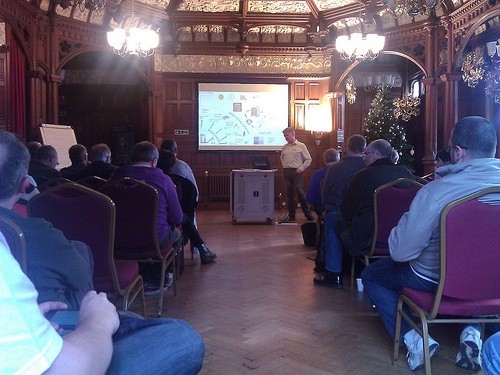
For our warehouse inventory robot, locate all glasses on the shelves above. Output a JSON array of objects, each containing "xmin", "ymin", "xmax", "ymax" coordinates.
[
  {"xmin": 362, "ymin": 152, "xmax": 379, "ymax": 156},
  {"xmin": 55, "ymin": 162, "xmax": 60, "ymax": 166}
]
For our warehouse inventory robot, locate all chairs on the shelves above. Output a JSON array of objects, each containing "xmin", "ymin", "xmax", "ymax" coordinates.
[
  {"xmin": 349, "ymin": 177, "xmax": 426, "ymax": 295},
  {"xmin": 24, "ymin": 181, "xmax": 146, "ymax": 322},
  {"xmin": 392, "ymin": 186, "xmax": 500, "ymax": 375},
  {"xmin": 167, "ymin": 173, "xmax": 200, "ymax": 279},
  {"xmin": 100, "ymin": 176, "xmax": 180, "ymax": 318}
]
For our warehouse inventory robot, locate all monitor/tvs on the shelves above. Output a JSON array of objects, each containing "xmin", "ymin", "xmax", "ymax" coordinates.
[{"xmin": 251, "ymin": 156, "xmax": 272, "ymax": 170}]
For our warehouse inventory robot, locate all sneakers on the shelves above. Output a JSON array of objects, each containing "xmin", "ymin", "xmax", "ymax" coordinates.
[
  {"xmin": 144, "ymin": 272, "xmax": 173, "ymax": 296},
  {"xmin": 403, "ymin": 333, "xmax": 440, "ymax": 370},
  {"xmin": 455, "ymin": 326, "xmax": 482, "ymax": 371}
]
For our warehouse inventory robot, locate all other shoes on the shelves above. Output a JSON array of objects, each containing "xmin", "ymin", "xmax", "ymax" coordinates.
[{"xmin": 307, "ymin": 250, "xmax": 316, "ymax": 260}]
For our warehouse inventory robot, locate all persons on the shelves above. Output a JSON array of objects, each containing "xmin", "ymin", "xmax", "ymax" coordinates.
[
  {"xmin": 25, "ymin": 146, "xmax": 63, "ymax": 192},
  {"xmin": 312, "ymin": 134, "xmax": 369, "ymax": 288},
  {"xmin": 57, "ymin": 144, "xmax": 92, "ymax": 185},
  {"xmin": 335, "ymin": 138, "xmax": 416, "ymax": 289},
  {"xmin": 434, "ymin": 146, "xmax": 453, "ymax": 172},
  {"xmin": 391, "ymin": 150, "xmax": 400, "ymax": 166},
  {"xmin": 75, "ymin": 144, "xmax": 121, "ymax": 185},
  {"xmin": 360, "ymin": 116, "xmax": 500, "ymax": 370},
  {"xmin": 277, "ymin": 126, "xmax": 315, "ymax": 223},
  {"xmin": 481, "ymin": 332, "xmax": 500, "ymax": 375},
  {"xmin": 306, "ymin": 147, "xmax": 341, "ymax": 253},
  {"xmin": 0, "ymin": 130, "xmax": 206, "ymax": 375},
  {"xmin": 25, "ymin": 141, "xmax": 42, "ymax": 157},
  {"xmin": 107, "ymin": 134, "xmax": 218, "ymax": 269}
]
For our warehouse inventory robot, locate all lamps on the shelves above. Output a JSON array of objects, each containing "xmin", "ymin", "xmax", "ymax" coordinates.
[
  {"xmin": 105, "ymin": 0, "xmax": 161, "ymax": 58},
  {"xmin": 334, "ymin": 0, "xmax": 386, "ymax": 62}
]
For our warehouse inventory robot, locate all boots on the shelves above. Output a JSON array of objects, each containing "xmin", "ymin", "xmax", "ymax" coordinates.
[{"xmin": 198, "ymin": 242, "xmax": 217, "ymax": 264}]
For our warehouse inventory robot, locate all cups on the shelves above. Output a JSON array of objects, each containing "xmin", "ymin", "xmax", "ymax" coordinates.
[{"xmin": 356, "ymin": 278, "xmax": 365, "ymax": 291}]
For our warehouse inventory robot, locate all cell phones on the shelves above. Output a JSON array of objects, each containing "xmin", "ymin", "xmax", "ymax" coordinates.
[{"xmin": 43, "ymin": 309, "xmax": 79, "ymax": 330}]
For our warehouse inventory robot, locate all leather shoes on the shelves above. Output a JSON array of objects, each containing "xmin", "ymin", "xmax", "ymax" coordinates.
[
  {"xmin": 314, "ymin": 275, "xmax": 344, "ymax": 289},
  {"xmin": 282, "ymin": 214, "xmax": 316, "ymax": 221}
]
